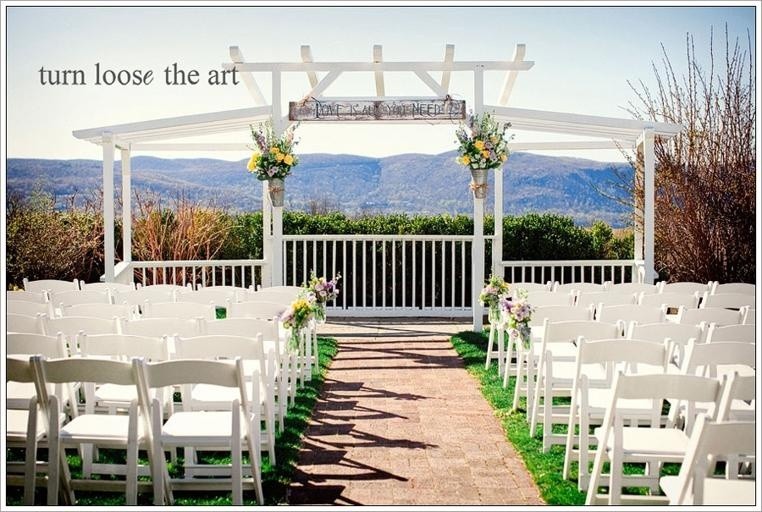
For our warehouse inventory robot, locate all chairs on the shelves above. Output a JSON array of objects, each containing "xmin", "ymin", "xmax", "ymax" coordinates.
[
  {"xmin": 6, "ymin": 355, "xmax": 77, "ymax": 505},
  {"xmin": 573, "ymin": 289, "xmax": 635, "ymax": 307},
  {"xmin": 23, "ymin": 277, "xmax": 79, "ymax": 291},
  {"xmin": 7, "ymin": 311, "xmax": 45, "ymax": 333},
  {"xmin": 115, "ymin": 290, "xmax": 172, "ymax": 306},
  {"xmin": 54, "ymin": 287, "xmax": 111, "ymax": 306},
  {"xmin": 638, "ymin": 291, "xmax": 700, "ymax": 309},
  {"xmin": 485, "ymin": 281, "xmax": 552, "ymax": 371},
  {"xmin": 584, "ymin": 372, "xmax": 723, "ymax": 506},
  {"xmin": 121, "ymin": 315, "xmax": 196, "ymax": 336},
  {"xmin": 228, "ymin": 298, "xmax": 297, "ymax": 408},
  {"xmin": 136, "ymin": 282, "xmax": 192, "ymax": 297},
  {"xmin": 513, "ymin": 304, "xmax": 595, "ymax": 421},
  {"xmin": 711, "ymin": 280, "xmax": 754, "ymax": 293},
  {"xmin": 37, "ymin": 353, "xmax": 174, "ymax": 504},
  {"xmin": 742, "ymin": 305, "xmax": 755, "ymax": 324},
  {"xmin": 239, "ymin": 292, "xmax": 307, "ymax": 390},
  {"xmin": 79, "ymin": 280, "xmax": 132, "ymax": 295},
  {"xmin": 145, "ymin": 299, "xmax": 217, "ymax": 318},
  {"xmin": 44, "ymin": 314, "xmax": 118, "ymax": 334},
  {"xmin": 6, "ymin": 332, "xmax": 67, "ymax": 361},
  {"xmin": 659, "ymin": 412, "xmax": 757, "ymax": 505},
  {"xmin": 529, "ymin": 319, "xmax": 623, "ymax": 454},
  {"xmin": 706, "ymin": 322, "xmax": 756, "ymax": 344},
  {"xmin": 61, "ymin": 301, "xmax": 136, "ymax": 317},
  {"xmin": 555, "ymin": 281, "xmax": 604, "ymax": 293},
  {"xmin": 719, "ymin": 370, "xmax": 757, "ymax": 478},
  {"xmin": 196, "ymin": 283, "xmax": 254, "ymax": 300},
  {"xmin": 630, "ymin": 319, "xmax": 701, "ymax": 361},
  {"xmin": 176, "ymin": 292, "xmax": 236, "ymax": 303},
  {"xmin": 701, "ymin": 291, "xmax": 755, "ymax": 309},
  {"xmin": 563, "ymin": 336, "xmax": 667, "ymax": 481},
  {"xmin": 598, "ymin": 302, "xmax": 668, "ymax": 320},
  {"xmin": 198, "ymin": 316, "xmax": 285, "ymax": 434},
  {"xmin": 79, "ymin": 331, "xmax": 177, "ymax": 477},
  {"xmin": 678, "ymin": 339, "xmax": 756, "ymax": 435},
  {"xmin": 132, "ymin": 357, "xmax": 264, "ymax": 505},
  {"xmin": 679, "ymin": 305, "xmax": 744, "ymax": 329},
  {"xmin": 498, "ymin": 291, "xmax": 579, "ymax": 389},
  {"xmin": 8, "ymin": 291, "xmax": 51, "ymax": 316},
  {"xmin": 258, "ymin": 284, "xmax": 320, "ymax": 382},
  {"xmin": 660, "ymin": 280, "xmax": 713, "ymax": 295},
  {"xmin": 174, "ymin": 334, "xmax": 276, "ymax": 467},
  {"xmin": 607, "ymin": 280, "xmax": 662, "ymax": 293}
]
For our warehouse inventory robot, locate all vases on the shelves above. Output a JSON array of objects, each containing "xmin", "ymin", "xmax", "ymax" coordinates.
[
  {"xmin": 270, "ymin": 177, "xmax": 286, "ymax": 208},
  {"xmin": 472, "ymin": 169, "xmax": 488, "ymax": 198}
]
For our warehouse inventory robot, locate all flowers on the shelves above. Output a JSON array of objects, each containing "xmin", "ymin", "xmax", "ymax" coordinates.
[
  {"xmin": 245, "ymin": 115, "xmax": 301, "ymax": 181},
  {"xmin": 455, "ymin": 108, "xmax": 515, "ymax": 169},
  {"xmin": 479, "ymin": 273, "xmax": 507, "ymax": 316},
  {"xmin": 282, "ymin": 298, "xmax": 324, "ymax": 350},
  {"xmin": 506, "ymin": 289, "xmax": 534, "ymax": 347},
  {"xmin": 305, "ymin": 272, "xmax": 341, "ymax": 319}
]
